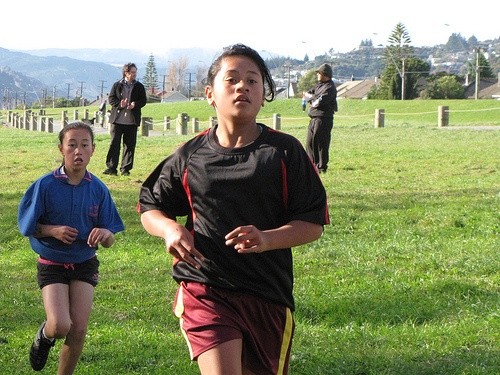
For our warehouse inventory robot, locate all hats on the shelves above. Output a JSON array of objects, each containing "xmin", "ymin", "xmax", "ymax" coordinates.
[{"xmin": 315, "ymin": 64, "xmax": 332, "ymax": 77}]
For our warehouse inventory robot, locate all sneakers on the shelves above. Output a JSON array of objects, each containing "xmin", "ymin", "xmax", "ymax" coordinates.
[{"xmin": 30, "ymin": 322, "xmax": 56, "ymax": 371}]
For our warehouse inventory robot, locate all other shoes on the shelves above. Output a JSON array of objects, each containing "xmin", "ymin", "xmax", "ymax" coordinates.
[
  {"xmin": 102, "ymin": 169, "xmax": 118, "ymax": 174},
  {"xmin": 121, "ymin": 171, "xmax": 130, "ymax": 176}
]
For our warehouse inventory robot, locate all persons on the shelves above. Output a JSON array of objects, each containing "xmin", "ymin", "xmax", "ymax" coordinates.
[
  {"xmin": 17, "ymin": 120, "xmax": 125, "ymax": 375},
  {"xmin": 303, "ymin": 64, "xmax": 338, "ymax": 172},
  {"xmin": 102, "ymin": 62, "xmax": 147, "ymax": 176},
  {"xmin": 138, "ymin": 44, "xmax": 331, "ymax": 375}
]
